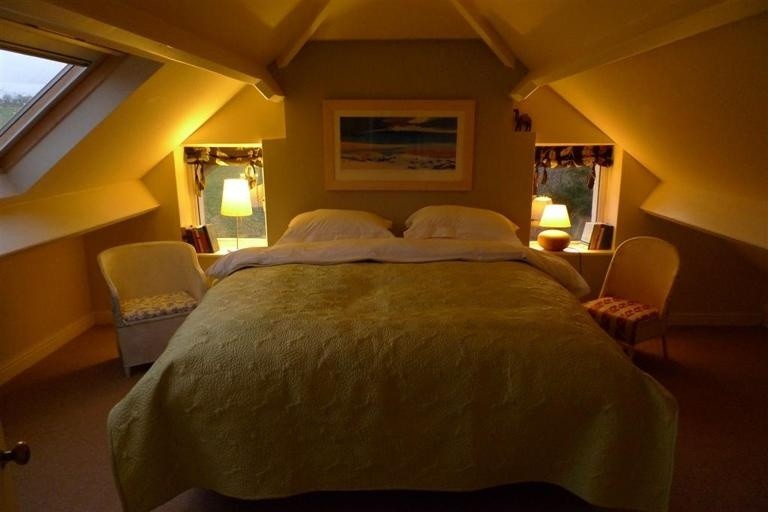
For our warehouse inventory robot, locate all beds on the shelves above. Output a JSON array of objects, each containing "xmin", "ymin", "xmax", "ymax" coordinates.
[{"xmin": 107, "ymin": 205, "xmax": 680, "ymax": 512}]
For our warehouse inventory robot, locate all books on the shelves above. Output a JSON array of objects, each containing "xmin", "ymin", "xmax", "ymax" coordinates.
[
  {"xmin": 578, "ymin": 221, "xmax": 614, "ymax": 250},
  {"xmin": 180, "ymin": 224, "xmax": 220, "ymax": 253}
]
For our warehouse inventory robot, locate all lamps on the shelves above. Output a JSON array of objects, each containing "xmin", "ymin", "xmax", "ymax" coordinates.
[
  {"xmin": 531, "ymin": 197, "xmax": 553, "ymax": 239},
  {"xmin": 221, "ymin": 178, "xmax": 253, "ymax": 252},
  {"xmin": 537, "ymin": 205, "xmax": 572, "ymax": 251}
]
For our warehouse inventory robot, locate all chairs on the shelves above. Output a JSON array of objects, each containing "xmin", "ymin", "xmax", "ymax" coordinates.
[
  {"xmin": 582, "ymin": 236, "xmax": 680, "ymax": 362},
  {"xmin": 96, "ymin": 241, "xmax": 209, "ymax": 378}
]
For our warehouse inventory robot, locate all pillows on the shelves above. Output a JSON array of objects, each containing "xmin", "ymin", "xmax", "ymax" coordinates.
[
  {"xmin": 403, "ymin": 205, "xmax": 523, "ymax": 241},
  {"xmin": 274, "ymin": 208, "xmax": 396, "ymax": 245}
]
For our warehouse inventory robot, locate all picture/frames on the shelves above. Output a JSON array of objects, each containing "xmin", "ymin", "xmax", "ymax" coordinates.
[{"xmin": 322, "ymin": 99, "xmax": 476, "ymax": 192}]
